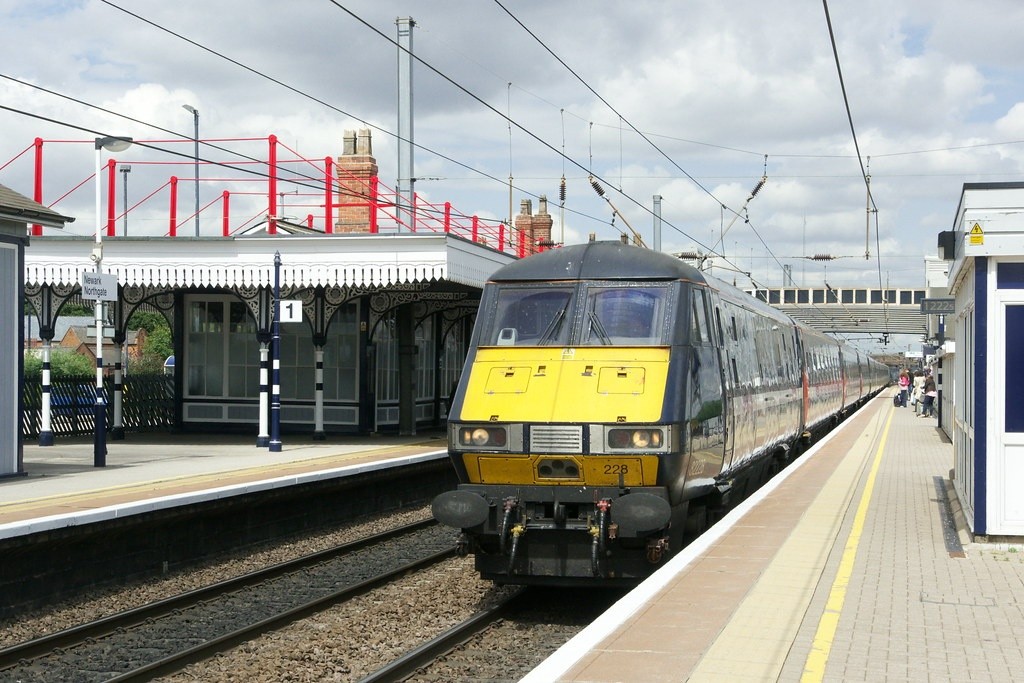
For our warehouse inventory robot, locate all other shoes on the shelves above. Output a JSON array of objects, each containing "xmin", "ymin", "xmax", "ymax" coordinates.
[{"xmin": 918, "ymin": 414, "xmax": 926, "ymax": 417}]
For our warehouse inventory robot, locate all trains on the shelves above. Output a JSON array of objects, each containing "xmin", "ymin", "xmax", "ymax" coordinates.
[{"xmin": 430, "ymin": 232, "xmax": 895, "ymax": 588}]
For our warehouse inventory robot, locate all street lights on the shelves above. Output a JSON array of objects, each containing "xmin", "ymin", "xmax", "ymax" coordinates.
[
  {"xmin": 91, "ymin": 136, "xmax": 133, "ymax": 468},
  {"xmin": 182, "ymin": 102, "xmax": 200, "ymax": 236}
]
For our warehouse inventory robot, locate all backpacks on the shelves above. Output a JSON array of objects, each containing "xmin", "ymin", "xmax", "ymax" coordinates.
[{"xmin": 894, "ymin": 396, "xmax": 900, "ymax": 407}]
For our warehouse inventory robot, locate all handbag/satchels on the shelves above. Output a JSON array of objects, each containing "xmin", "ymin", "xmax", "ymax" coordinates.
[
  {"xmin": 926, "ymin": 391, "xmax": 937, "ymax": 397},
  {"xmin": 899, "ymin": 383, "xmax": 908, "ymax": 390}
]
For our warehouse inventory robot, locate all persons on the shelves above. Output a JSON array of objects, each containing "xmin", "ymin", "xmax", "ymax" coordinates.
[
  {"xmin": 919, "ymin": 375, "xmax": 937, "ymax": 419},
  {"xmin": 898, "ymin": 368, "xmax": 914, "ymax": 407},
  {"xmin": 910, "ymin": 367, "xmax": 932, "ymax": 411}
]
